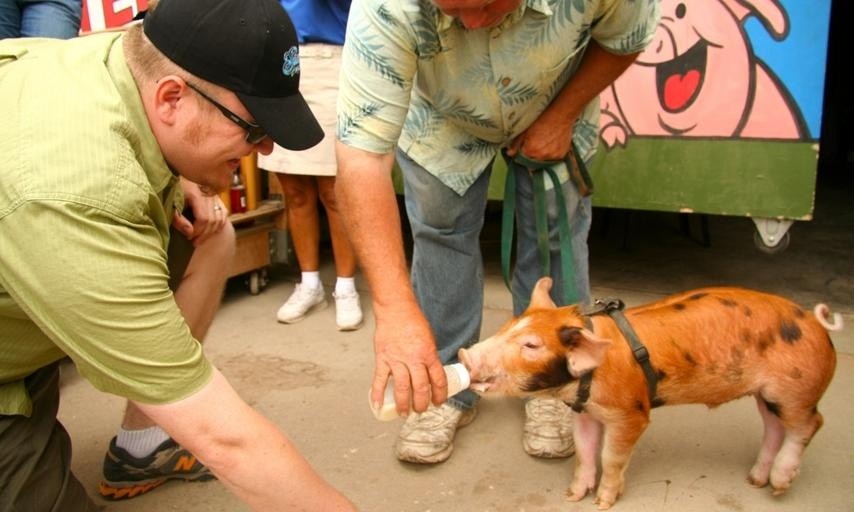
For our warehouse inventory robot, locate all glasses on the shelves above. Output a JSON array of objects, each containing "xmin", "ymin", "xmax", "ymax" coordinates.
[{"xmin": 185, "ymin": 85, "xmax": 268, "ymax": 145}]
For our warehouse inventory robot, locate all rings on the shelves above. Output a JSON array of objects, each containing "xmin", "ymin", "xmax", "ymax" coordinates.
[{"xmin": 213, "ymin": 206, "xmax": 221, "ymax": 210}]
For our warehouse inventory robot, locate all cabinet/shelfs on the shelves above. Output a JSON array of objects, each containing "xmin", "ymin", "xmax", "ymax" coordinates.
[{"xmin": 167, "ymin": 143, "xmax": 286, "ymax": 293}]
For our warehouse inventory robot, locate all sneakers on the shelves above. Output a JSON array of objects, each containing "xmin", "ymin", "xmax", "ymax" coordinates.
[
  {"xmin": 333, "ymin": 282, "xmax": 365, "ymax": 331},
  {"xmin": 99, "ymin": 434, "xmax": 221, "ymax": 500},
  {"xmin": 524, "ymin": 399, "xmax": 576, "ymax": 459},
  {"xmin": 277, "ymin": 280, "xmax": 329, "ymax": 324},
  {"xmin": 395, "ymin": 402, "xmax": 478, "ymax": 464}
]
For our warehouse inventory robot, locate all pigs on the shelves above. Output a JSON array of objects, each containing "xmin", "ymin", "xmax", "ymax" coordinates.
[{"xmin": 457, "ymin": 276, "xmax": 845, "ymax": 510}]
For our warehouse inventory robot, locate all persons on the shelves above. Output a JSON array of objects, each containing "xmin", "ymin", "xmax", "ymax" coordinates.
[
  {"xmin": 0, "ymin": 1, "xmax": 82, "ymax": 40},
  {"xmin": 0, "ymin": 1, "xmax": 363, "ymax": 511},
  {"xmin": 331, "ymin": 0, "xmax": 660, "ymax": 466},
  {"xmin": 257, "ymin": 1, "xmax": 364, "ymax": 331}
]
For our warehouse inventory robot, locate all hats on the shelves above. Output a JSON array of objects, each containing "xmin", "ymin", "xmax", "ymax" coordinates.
[{"xmin": 142, "ymin": 1, "xmax": 324, "ymax": 150}]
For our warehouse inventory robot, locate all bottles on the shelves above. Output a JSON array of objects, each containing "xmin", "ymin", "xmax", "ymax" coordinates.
[
  {"xmin": 230, "ymin": 185, "xmax": 249, "ymax": 216},
  {"xmin": 365, "ymin": 360, "xmax": 473, "ymax": 422}
]
